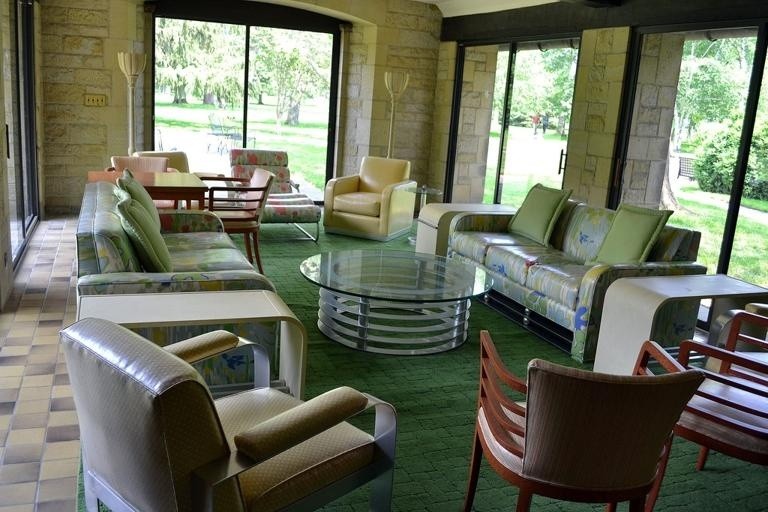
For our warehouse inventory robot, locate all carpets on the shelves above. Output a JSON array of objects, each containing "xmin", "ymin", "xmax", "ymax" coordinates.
[{"xmin": 76, "ymin": 211, "xmax": 768, "ymax": 512}]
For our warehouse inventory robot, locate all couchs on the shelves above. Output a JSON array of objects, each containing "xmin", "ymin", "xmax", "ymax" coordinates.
[
  {"xmin": 322, "ymin": 156, "xmax": 418, "ymax": 242},
  {"xmin": 133, "ymin": 151, "xmax": 224, "ymax": 209},
  {"xmin": 75, "ymin": 181, "xmax": 279, "ymax": 386},
  {"xmin": 417, "ymin": 197, "xmax": 708, "ymax": 365}
]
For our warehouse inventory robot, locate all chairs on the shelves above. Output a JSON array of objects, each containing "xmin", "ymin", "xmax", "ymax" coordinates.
[
  {"xmin": 194, "ymin": 167, "xmax": 276, "ymax": 274},
  {"xmin": 704, "ymin": 303, "xmax": 768, "ymax": 373},
  {"xmin": 228, "ymin": 148, "xmax": 321, "ymax": 242},
  {"xmin": 645, "ymin": 312, "xmax": 768, "ymax": 511},
  {"xmin": 57, "ymin": 316, "xmax": 398, "ymax": 512},
  {"xmin": 106, "ymin": 156, "xmax": 181, "ymax": 209},
  {"xmin": 462, "ymin": 329, "xmax": 705, "ymax": 512}
]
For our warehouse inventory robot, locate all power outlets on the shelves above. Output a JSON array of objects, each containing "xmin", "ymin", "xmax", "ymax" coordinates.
[{"xmin": 85, "ymin": 94, "xmax": 106, "ymax": 107}]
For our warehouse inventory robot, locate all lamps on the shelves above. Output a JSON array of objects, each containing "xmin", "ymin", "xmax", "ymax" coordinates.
[
  {"xmin": 384, "ymin": 71, "xmax": 410, "ymax": 159},
  {"xmin": 118, "ymin": 52, "xmax": 148, "ymax": 157}
]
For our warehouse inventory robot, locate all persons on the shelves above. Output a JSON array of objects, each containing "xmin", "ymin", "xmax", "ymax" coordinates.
[
  {"xmin": 540, "ymin": 113, "xmax": 549, "ymax": 134},
  {"xmin": 531, "ymin": 112, "xmax": 540, "ymax": 135}
]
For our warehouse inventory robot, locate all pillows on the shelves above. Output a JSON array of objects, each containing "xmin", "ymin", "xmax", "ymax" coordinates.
[
  {"xmin": 507, "ymin": 183, "xmax": 574, "ymax": 247},
  {"xmin": 584, "ymin": 202, "xmax": 674, "ymax": 267},
  {"xmin": 116, "ymin": 168, "xmax": 162, "ymax": 232},
  {"xmin": 113, "ymin": 184, "xmax": 173, "ymax": 273}
]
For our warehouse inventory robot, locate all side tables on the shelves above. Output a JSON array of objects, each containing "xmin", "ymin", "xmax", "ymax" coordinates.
[
  {"xmin": 404, "ymin": 184, "xmax": 442, "ymax": 245},
  {"xmin": 75, "ymin": 289, "xmax": 308, "ymax": 400},
  {"xmin": 593, "ymin": 273, "xmax": 768, "ymax": 376}
]
